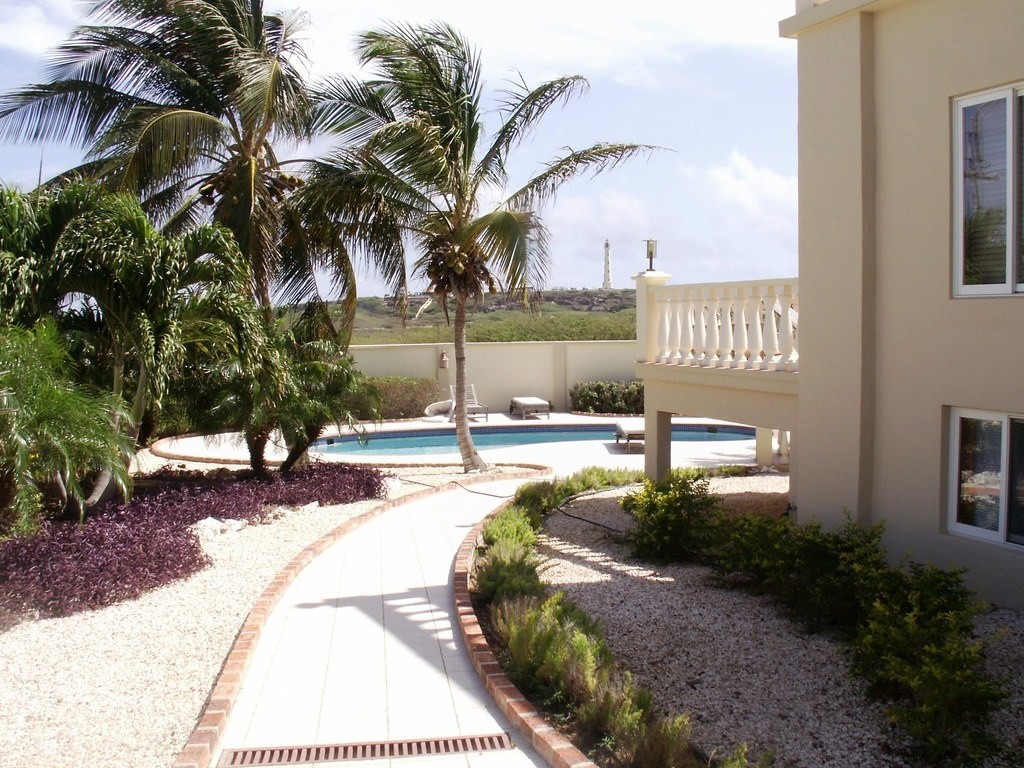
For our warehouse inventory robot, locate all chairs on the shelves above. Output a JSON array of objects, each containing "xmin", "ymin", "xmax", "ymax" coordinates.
[{"xmin": 447, "ymin": 382, "xmax": 489, "ymax": 422}]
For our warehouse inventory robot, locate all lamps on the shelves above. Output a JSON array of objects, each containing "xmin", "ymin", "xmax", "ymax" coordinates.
[{"xmin": 644, "ymin": 237, "xmax": 659, "ymax": 272}]
